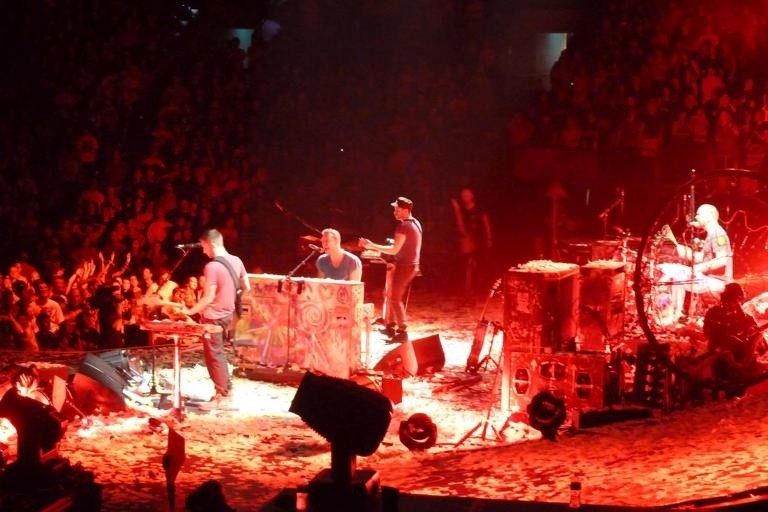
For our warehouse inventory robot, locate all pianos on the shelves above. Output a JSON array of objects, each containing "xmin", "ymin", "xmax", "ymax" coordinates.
[
  {"xmin": 139, "ymin": 321, "xmax": 223, "ymax": 334},
  {"xmin": 232, "ymin": 274, "xmax": 375, "ymax": 380}
]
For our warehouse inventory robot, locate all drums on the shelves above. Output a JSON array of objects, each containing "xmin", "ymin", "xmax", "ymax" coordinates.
[
  {"xmin": 594, "ymin": 240, "xmax": 620, "ymax": 263},
  {"xmin": 566, "ymin": 242, "xmax": 591, "ymax": 276}
]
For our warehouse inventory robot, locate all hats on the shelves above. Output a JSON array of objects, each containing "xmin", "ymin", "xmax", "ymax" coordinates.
[{"xmin": 390, "ymin": 197, "xmax": 412, "ymax": 210}]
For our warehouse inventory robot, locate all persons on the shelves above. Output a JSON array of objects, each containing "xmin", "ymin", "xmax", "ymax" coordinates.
[
  {"xmin": 702, "ymin": 281, "xmax": 768, "ymax": 392},
  {"xmin": 179, "ymin": 227, "xmax": 251, "ymax": 412},
  {"xmin": 0, "ymin": 0, "xmax": 768, "ymax": 354}
]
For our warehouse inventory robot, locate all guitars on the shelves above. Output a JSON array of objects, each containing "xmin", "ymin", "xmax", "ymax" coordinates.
[
  {"xmin": 450, "ymin": 197, "xmax": 485, "ymax": 263},
  {"xmin": 467, "ymin": 278, "xmax": 502, "ymax": 375}
]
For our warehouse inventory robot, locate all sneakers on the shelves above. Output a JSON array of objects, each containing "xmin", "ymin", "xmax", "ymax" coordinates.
[
  {"xmin": 198, "ymin": 393, "xmax": 231, "ymax": 411},
  {"xmin": 378, "ymin": 328, "xmax": 394, "ymax": 337},
  {"xmin": 386, "ymin": 332, "xmax": 408, "ymax": 343}
]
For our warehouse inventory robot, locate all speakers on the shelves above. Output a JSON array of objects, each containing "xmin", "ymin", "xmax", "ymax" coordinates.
[
  {"xmin": 98, "ymin": 349, "xmax": 129, "ymax": 369},
  {"xmin": 372, "ymin": 332, "xmax": 445, "ymax": 376},
  {"xmin": 73, "ymin": 353, "xmax": 124, "ymax": 396}
]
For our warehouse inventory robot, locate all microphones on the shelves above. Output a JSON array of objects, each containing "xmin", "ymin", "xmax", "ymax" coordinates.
[
  {"xmin": 175, "ymin": 241, "xmax": 202, "ymax": 251},
  {"xmin": 309, "ymin": 241, "xmax": 327, "ymax": 258}
]
